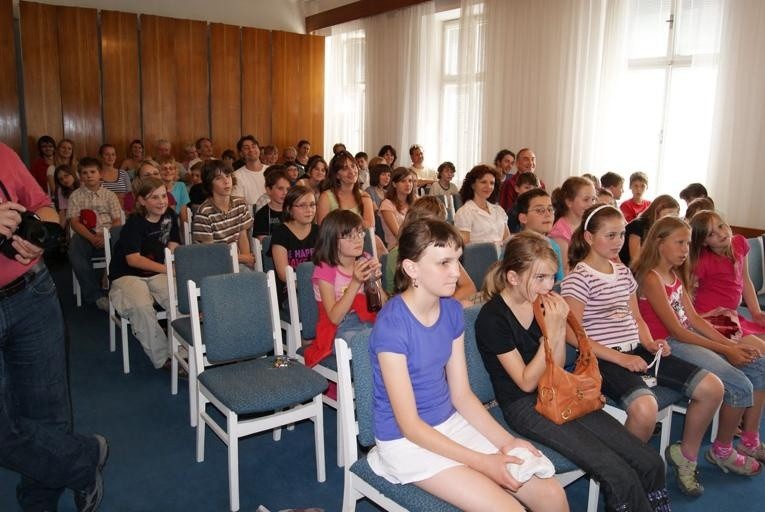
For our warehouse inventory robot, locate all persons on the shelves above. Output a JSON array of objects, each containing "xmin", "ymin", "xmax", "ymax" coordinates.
[
  {"xmin": 473, "ymin": 229, "xmax": 670, "ymax": 512},
  {"xmin": 686, "ymin": 209, "xmax": 765, "ymax": 358},
  {"xmin": 1, "ymin": 143, "xmax": 109, "ymax": 512},
  {"xmin": 303, "ymin": 162, "xmax": 712, "ymax": 411},
  {"xmin": 562, "ymin": 203, "xmax": 724, "ymax": 496},
  {"xmin": 32, "ymin": 135, "xmax": 546, "ymax": 373},
  {"xmin": 632, "ymin": 217, "xmax": 765, "ymax": 479},
  {"xmin": 364, "ymin": 217, "xmax": 571, "ymax": 512}
]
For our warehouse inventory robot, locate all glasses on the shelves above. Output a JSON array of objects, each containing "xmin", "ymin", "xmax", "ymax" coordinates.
[
  {"xmin": 338, "ymin": 232, "xmax": 366, "ymax": 241},
  {"xmin": 525, "ymin": 208, "xmax": 559, "ymax": 216},
  {"xmin": 290, "ymin": 202, "xmax": 318, "ymax": 210}
]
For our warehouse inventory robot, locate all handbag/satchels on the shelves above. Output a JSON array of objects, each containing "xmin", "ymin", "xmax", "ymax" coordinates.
[{"xmin": 534, "ymin": 352, "xmax": 606, "ymax": 425}]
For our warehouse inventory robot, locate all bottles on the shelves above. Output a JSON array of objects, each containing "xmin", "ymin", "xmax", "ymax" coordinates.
[{"xmin": 364, "ymin": 266, "xmax": 382, "ymax": 312}]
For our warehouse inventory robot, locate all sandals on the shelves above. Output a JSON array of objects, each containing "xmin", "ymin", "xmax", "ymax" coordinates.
[
  {"xmin": 706, "ymin": 444, "xmax": 760, "ymax": 477},
  {"xmin": 737, "ymin": 438, "xmax": 765, "ymax": 459}
]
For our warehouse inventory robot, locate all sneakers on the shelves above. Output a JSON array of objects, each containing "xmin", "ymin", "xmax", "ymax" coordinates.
[
  {"xmin": 164, "ymin": 357, "xmax": 187, "ymax": 377},
  {"xmin": 663, "ymin": 440, "xmax": 706, "ymax": 498},
  {"xmin": 74, "ymin": 433, "xmax": 107, "ymax": 512},
  {"xmin": 95, "ymin": 296, "xmax": 110, "ymax": 313}
]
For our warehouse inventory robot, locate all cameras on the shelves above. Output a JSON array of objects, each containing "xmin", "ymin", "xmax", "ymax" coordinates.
[{"xmin": 0, "ymin": 210, "xmax": 64, "ymax": 261}]
